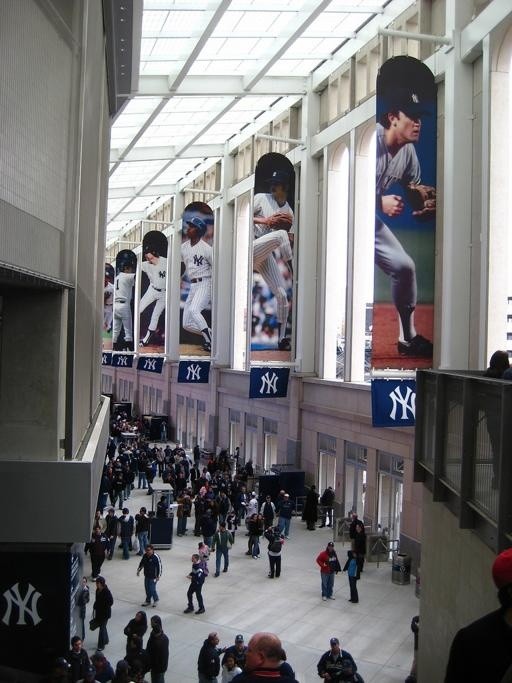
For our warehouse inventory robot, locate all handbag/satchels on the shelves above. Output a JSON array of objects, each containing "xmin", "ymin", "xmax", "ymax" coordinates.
[
  {"xmin": 133, "ymin": 538, "xmax": 140, "ymax": 552},
  {"xmin": 90, "ymin": 617, "xmax": 100, "ymax": 630}
]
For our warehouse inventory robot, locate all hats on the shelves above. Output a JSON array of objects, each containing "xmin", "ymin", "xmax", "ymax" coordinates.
[
  {"xmin": 328, "ymin": 541, "xmax": 334, "ymax": 546},
  {"xmin": 236, "ymin": 634, "xmax": 244, "ymax": 642},
  {"xmin": 265, "ymin": 168, "xmax": 290, "ymax": 185},
  {"xmin": 491, "ymin": 549, "xmax": 512, "ymax": 587},
  {"xmin": 390, "ymin": 84, "xmax": 433, "ymax": 116},
  {"xmin": 330, "ymin": 637, "xmax": 339, "ymax": 644},
  {"xmin": 55, "ymin": 657, "xmax": 72, "ymax": 669}
]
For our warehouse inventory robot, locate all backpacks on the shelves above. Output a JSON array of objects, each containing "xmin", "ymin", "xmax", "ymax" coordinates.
[{"xmin": 269, "ymin": 533, "xmax": 281, "ymax": 552}]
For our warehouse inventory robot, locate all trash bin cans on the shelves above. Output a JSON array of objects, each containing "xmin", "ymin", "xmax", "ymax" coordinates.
[
  {"xmin": 391, "ymin": 553, "xmax": 411, "ymax": 584},
  {"xmin": 415, "ymin": 567, "xmax": 422, "ymax": 598},
  {"xmin": 411, "ymin": 616, "xmax": 419, "ymax": 658}
]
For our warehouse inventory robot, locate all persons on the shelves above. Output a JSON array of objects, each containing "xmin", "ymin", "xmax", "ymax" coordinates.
[
  {"xmin": 220, "ymin": 632, "xmax": 250, "ymax": 672},
  {"xmin": 90, "ymin": 576, "xmax": 114, "ymax": 650},
  {"xmin": 80, "ymin": 576, "xmax": 89, "ymax": 642},
  {"xmin": 134, "ymin": 544, "xmax": 163, "ymax": 607},
  {"xmin": 113, "ymin": 260, "xmax": 135, "ymax": 351},
  {"xmin": 302, "ymin": 484, "xmax": 319, "ymax": 530},
  {"xmin": 141, "ymin": 246, "xmax": 166, "ymax": 346},
  {"xmin": 251, "ymin": 171, "xmax": 296, "ymax": 354},
  {"xmin": 182, "ymin": 216, "xmax": 212, "ymax": 353},
  {"xmin": 316, "ymin": 638, "xmax": 364, "ymax": 682},
  {"xmin": 218, "ymin": 652, "xmax": 243, "ymax": 682},
  {"xmin": 50, "ymin": 612, "xmax": 169, "ymax": 682},
  {"xmin": 182, "ymin": 554, "xmax": 208, "ymax": 614},
  {"xmin": 104, "ymin": 270, "xmax": 113, "ymax": 330},
  {"xmin": 444, "ymin": 547, "xmax": 512, "ymax": 682},
  {"xmin": 197, "ymin": 631, "xmax": 228, "ymax": 682},
  {"xmin": 372, "ymin": 90, "xmax": 436, "ymax": 361},
  {"xmin": 229, "ymin": 631, "xmax": 298, "ymax": 682},
  {"xmin": 355, "ymin": 523, "xmax": 365, "ymax": 572},
  {"xmin": 315, "ymin": 542, "xmax": 341, "ymax": 600},
  {"xmin": 319, "ymin": 486, "xmax": 334, "ymax": 528},
  {"xmin": 349, "ymin": 512, "xmax": 365, "ymax": 549},
  {"xmin": 343, "ymin": 549, "xmax": 358, "ymax": 603},
  {"xmin": 481, "ymin": 350, "xmax": 512, "ymax": 491},
  {"xmin": 87, "ymin": 413, "xmax": 294, "ymax": 582}
]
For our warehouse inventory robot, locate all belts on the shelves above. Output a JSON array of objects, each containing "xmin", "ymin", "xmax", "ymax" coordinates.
[
  {"xmin": 116, "ymin": 300, "xmax": 126, "ymax": 303},
  {"xmin": 106, "ymin": 304, "xmax": 113, "ymax": 305},
  {"xmin": 152, "ymin": 285, "xmax": 166, "ymax": 291},
  {"xmin": 191, "ymin": 277, "xmax": 203, "ymax": 282}
]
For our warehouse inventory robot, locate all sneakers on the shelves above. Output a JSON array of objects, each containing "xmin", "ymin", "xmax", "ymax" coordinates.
[
  {"xmin": 277, "ymin": 338, "xmax": 291, "ymax": 351},
  {"xmin": 97, "ymin": 646, "xmax": 105, "ymax": 650},
  {"xmin": 141, "ymin": 551, "xmax": 359, "ymax": 614},
  {"xmin": 113, "ymin": 340, "xmax": 211, "ymax": 351},
  {"xmin": 397, "ymin": 333, "xmax": 433, "ymax": 359}
]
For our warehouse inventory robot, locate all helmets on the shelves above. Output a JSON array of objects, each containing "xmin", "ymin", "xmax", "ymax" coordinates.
[
  {"xmin": 186, "ymin": 216, "xmax": 207, "ymax": 235},
  {"xmin": 143, "ymin": 245, "xmax": 154, "ymax": 254},
  {"xmin": 119, "ymin": 259, "xmax": 133, "ymax": 267},
  {"xmin": 105, "ymin": 262, "xmax": 115, "ymax": 279}
]
